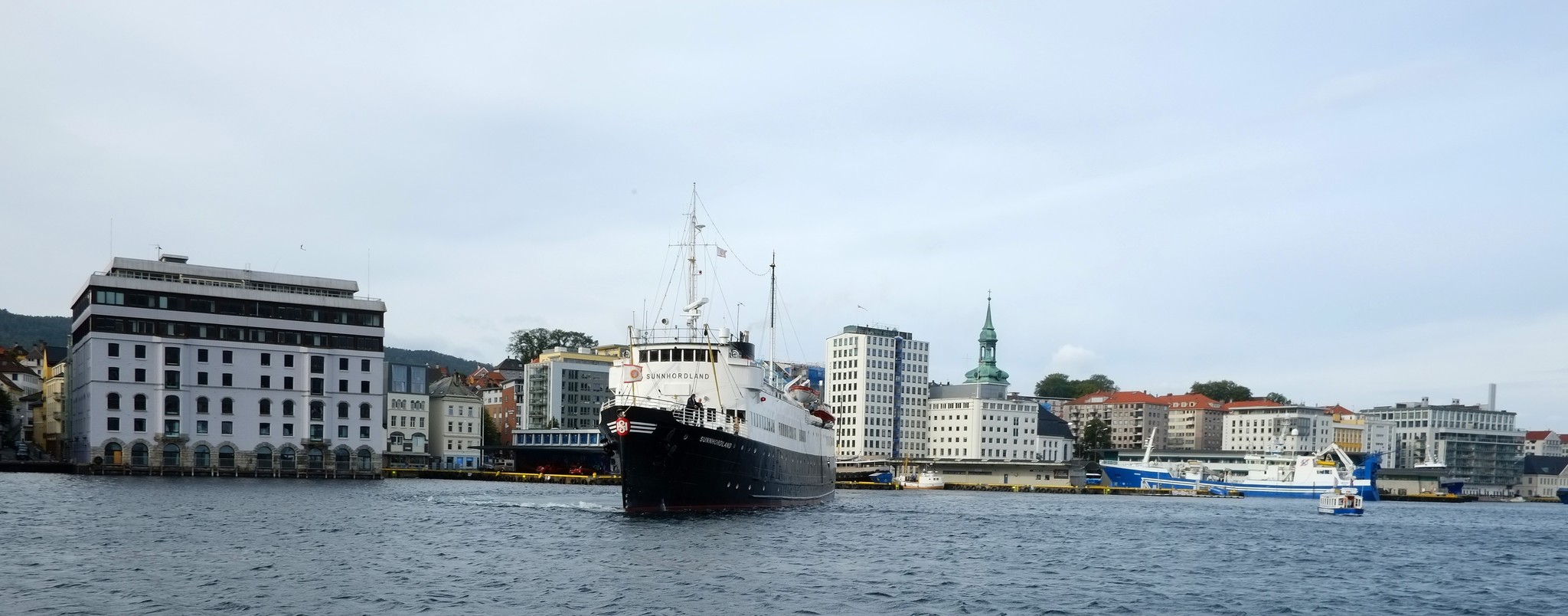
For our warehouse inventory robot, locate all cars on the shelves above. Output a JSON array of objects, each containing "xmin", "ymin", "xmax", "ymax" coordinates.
[
  {"xmin": 13, "ymin": 441, "xmax": 23, "ymax": 449},
  {"xmin": 478, "ymin": 464, "xmax": 494, "ymax": 471},
  {"xmin": 535, "ymin": 462, "xmax": 563, "ymax": 474},
  {"xmin": 16, "ymin": 444, "xmax": 28, "ymax": 451},
  {"xmin": 569, "ymin": 465, "xmax": 596, "ymax": 475},
  {"xmin": 16, "ymin": 449, "xmax": 29, "ymax": 460}
]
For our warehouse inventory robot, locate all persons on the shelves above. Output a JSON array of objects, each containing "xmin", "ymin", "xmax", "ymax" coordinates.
[
  {"xmin": 684, "ymin": 394, "xmax": 700, "ymax": 425},
  {"xmin": 694, "ymin": 398, "xmax": 704, "ymax": 428}
]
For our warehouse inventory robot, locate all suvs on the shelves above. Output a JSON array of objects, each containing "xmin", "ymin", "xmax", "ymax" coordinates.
[{"xmin": 494, "ymin": 462, "xmax": 513, "ymax": 472}]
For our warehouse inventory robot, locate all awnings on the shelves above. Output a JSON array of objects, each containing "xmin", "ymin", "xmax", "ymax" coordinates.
[{"xmin": 382, "ymin": 451, "xmax": 431, "ymax": 456}]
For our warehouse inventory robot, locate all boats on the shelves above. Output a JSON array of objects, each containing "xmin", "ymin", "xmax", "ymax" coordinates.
[
  {"xmin": 1414, "ymin": 444, "xmax": 1448, "ymax": 469},
  {"xmin": 788, "ymin": 385, "xmax": 819, "ymax": 405},
  {"xmin": 1317, "ymin": 476, "xmax": 1364, "ymax": 517},
  {"xmin": 1098, "ymin": 424, "xmax": 1383, "ymax": 501},
  {"xmin": 893, "ymin": 453, "xmax": 945, "ymax": 490},
  {"xmin": 811, "ymin": 409, "xmax": 836, "ymax": 425},
  {"xmin": 1510, "ymin": 496, "xmax": 1528, "ymax": 502},
  {"xmin": 1556, "ymin": 488, "xmax": 1568, "ymax": 504},
  {"xmin": 597, "ymin": 179, "xmax": 836, "ymax": 518},
  {"xmin": 1500, "ymin": 499, "xmax": 1511, "ymax": 502}
]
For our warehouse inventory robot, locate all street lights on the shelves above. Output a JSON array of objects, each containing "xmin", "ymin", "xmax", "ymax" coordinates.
[
  {"xmin": 1094, "ymin": 428, "xmax": 1108, "ymax": 462},
  {"xmin": 1079, "ymin": 425, "xmax": 1092, "ymax": 466}
]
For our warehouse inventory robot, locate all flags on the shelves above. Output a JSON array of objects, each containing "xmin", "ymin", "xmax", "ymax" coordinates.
[
  {"xmin": 717, "ymin": 247, "xmax": 727, "ymax": 258},
  {"xmin": 623, "ymin": 364, "xmax": 643, "ymax": 383}
]
